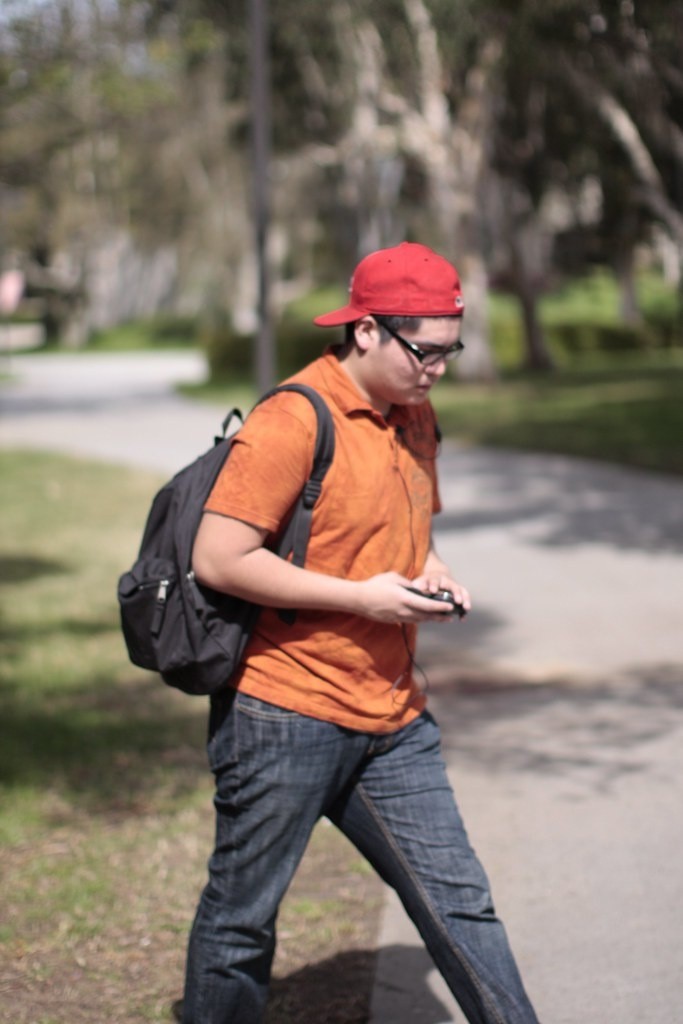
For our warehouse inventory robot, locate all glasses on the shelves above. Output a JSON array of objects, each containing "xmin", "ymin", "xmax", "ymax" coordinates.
[{"xmin": 375, "ymin": 319, "xmax": 464, "ymax": 366}]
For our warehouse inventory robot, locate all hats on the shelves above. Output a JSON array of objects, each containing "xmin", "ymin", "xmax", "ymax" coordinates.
[{"xmin": 314, "ymin": 242, "xmax": 464, "ymax": 326}]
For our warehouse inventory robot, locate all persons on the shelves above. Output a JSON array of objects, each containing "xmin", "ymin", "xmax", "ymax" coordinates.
[
  {"xmin": 173, "ymin": 244, "xmax": 539, "ymax": 1024},
  {"xmin": 24, "ymin": 248, "xmax": 67, "ymax": 337}
]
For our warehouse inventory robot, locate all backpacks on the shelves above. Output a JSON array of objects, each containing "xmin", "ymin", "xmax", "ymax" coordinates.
[{"xmin": 117, "ymin": 383, "xmax": 334, "ymax": 697}]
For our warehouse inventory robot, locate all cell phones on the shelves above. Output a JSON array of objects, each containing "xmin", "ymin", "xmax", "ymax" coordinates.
[{"xmin": 409, "ymin": 587, "xmax": 462, "ymax": 609}]
